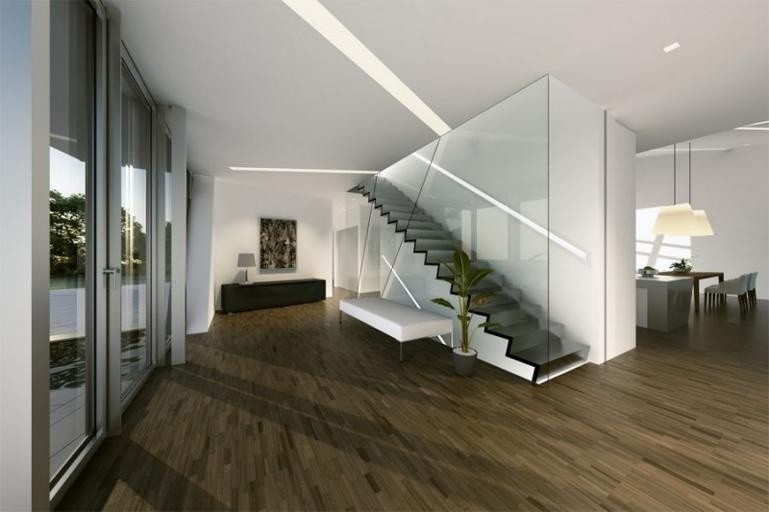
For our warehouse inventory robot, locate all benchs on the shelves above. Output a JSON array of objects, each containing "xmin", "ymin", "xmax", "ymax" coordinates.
[{"xmin": 337, "ymin": 295, "xmax": 455, "ymax": 361}]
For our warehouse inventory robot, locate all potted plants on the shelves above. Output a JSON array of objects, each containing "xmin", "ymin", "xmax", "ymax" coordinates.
[
  {"xmin": 668, "ymin": 257, "xmax": 692, "ymax": 274},
  {"xmin": 431, "ymin": 246, "xmax": 501, "ymax": 375}
]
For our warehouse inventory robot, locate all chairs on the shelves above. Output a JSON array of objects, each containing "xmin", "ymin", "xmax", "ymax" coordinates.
[{"xmin": 702, "ymin": 271, "xmax": 760, "ymax": 317}]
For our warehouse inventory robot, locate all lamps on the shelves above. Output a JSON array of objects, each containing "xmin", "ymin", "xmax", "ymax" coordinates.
[
  {"xmin": 236, "ymin": 253, "xmax": 258, "ymax": 286},
  {"xmin": 653, "ymin": 141, "xmax": 713, "ymax": 240}
]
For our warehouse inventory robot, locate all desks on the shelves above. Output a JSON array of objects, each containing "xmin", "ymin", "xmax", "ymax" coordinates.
[
  {"xmin": 658, "ymin": 271, "xmax": 726, "ymax": 313},
  {"xmin": 220, "ymin": 277, "xmax": 329, "ymax": 315}
]
